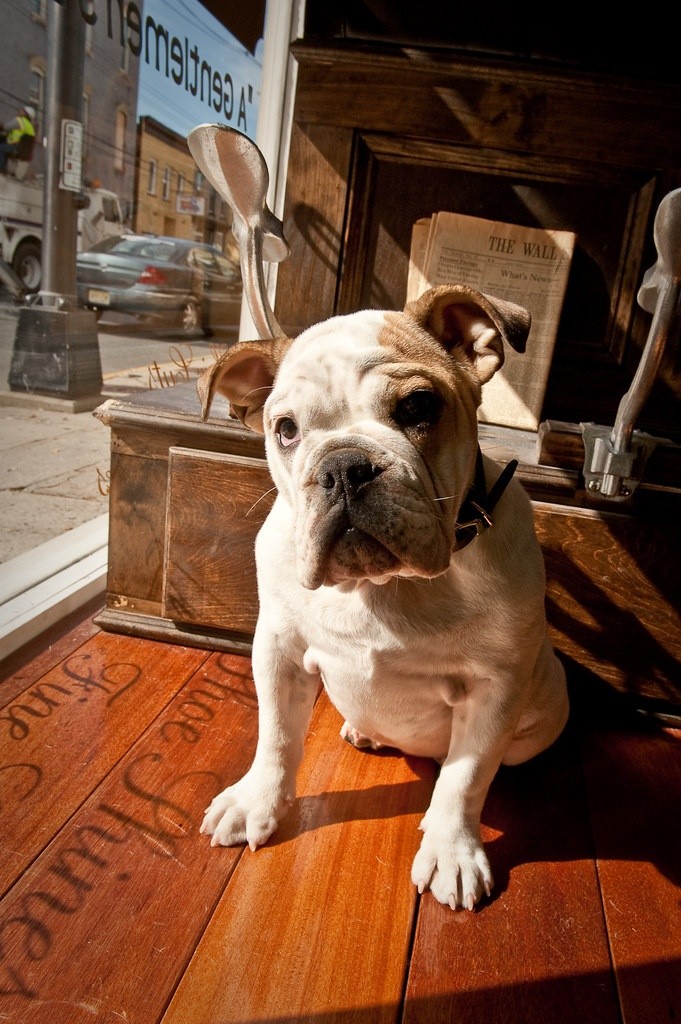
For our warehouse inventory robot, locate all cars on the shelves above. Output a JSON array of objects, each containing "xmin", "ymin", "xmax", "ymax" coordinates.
[{"xmin": 75, "ymin": 234, "xmax": 242, "ymax": 339}]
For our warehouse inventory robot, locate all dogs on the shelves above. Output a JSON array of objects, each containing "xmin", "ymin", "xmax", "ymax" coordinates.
[{"xmin": 198, "ymin": 282, "xmax": 569, "ymax": 912}]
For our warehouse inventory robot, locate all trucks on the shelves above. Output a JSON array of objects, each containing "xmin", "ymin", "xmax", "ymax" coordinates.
[{"xmin": 0, "ymin": 163, "xmax": 132, "ymax": 305}]
